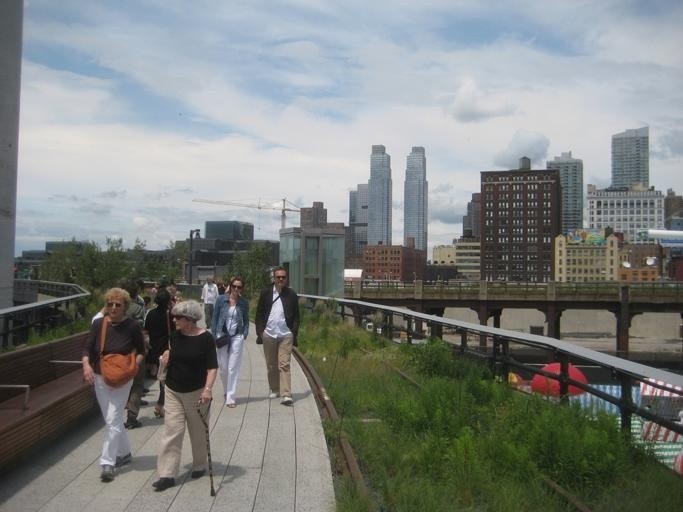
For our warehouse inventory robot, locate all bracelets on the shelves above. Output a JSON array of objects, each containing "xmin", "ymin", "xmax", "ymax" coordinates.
[{"xmin": 205, "ymin": 386, "xmax": 212, "ymax": 392}]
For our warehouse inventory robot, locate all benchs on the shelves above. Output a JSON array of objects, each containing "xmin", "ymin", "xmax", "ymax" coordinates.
[{"xmin": 0, "ymin": 319, "xmax": 152, "ymax": 477}]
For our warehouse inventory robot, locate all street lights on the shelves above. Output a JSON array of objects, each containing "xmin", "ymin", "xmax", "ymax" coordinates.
[
  {"xmin": 384, "ymin": 272, "xmax": 387, "ymax": 281},
  {"xmin": 411, "ymin": 271, "xmax": 416, "ymax": 286},
  {"xmin": 188, "ymin": 229, "xmax": 201, "ymax": 285},
  {"xmin": 388, "ymin": 271, "xmax": 392, "ymax": 286}
]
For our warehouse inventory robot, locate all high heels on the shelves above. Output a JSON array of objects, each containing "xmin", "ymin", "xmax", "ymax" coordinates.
[{"xmin": 153, "ymin": 405, "xmax": 165, "ymax": 419}]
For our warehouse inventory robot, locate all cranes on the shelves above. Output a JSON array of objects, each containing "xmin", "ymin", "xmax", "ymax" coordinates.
[{"xmin": 191, "ymin": 196, "xmax": 300, "ymax": 229}]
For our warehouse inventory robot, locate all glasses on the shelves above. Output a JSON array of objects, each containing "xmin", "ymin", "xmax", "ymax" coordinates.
[
  {"xmin": 173, "ymin": 314, "xmax": 185, "ymax": 321},
  {"xmin": 105, "ymin": 302, "xmax": 122, "ymax": 308},
  {"xmin": 231, "ymin": 284, "xmax": 243, "ymax": 290}
]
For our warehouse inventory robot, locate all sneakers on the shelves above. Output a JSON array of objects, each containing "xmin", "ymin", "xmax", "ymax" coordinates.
[
  {"xmin": 279, "ymin": 395, "xmax": 295, "ymax": 405},
  {"xmin": 100, "ymin": 464, "xmax": 115, "ymax": 480},
  {"xmin": 151, "ymin": 475, "xmax": 176, "ymax": 489},
  {"xmin": 140, "ymin": 387, "xmax": 150, "ymax": 407},
  {"xmin": 125, "ymin": 410, "xmax": 144, "ymax": 427},
  {"xmin": 192, "ymin": 469, "xmax": 206, "ymax": 479},
  {"xmin": 114, "ymin": 454, "xmax": 133, "ymax": 467},
  {"xmin": 267, "ymin": 391, "xmax": 282, "ymax": 398},
  {"xmin": 224, "ymin": 393, "xmax": 236, "ymax": 409}
]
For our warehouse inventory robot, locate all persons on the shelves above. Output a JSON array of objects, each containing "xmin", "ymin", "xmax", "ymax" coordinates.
[
  {"xmin": 210, "ymin": 277, "xmax": 249, "ymax": 409},
  {"xmin": 81, "ymin": 287, "xmax": 146, "ymax": 480},
  {"xmin": 202, "ymin": 274, "xmax": 242, "ymax": 329},
  {"xmin": 92, "ymin": 275, "xmax": 183, "ymax": 427},
  {"xmin": 254, "ymin": 266, "xmax": 300, "ymax": 405},
  {"xmin": 151, "ymin": 299, "xmax": 218, "ymax": 489}
]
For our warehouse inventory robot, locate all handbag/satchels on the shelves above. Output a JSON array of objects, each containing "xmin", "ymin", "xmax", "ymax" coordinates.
[
  {"xmin": 157, "ymin": 355, "xmax": 167, "ymax": 381},
  {"xmin": 214, "ymin": 335, "xmax": 231, "ymax": 348},
  {"xmin": 100, "ymin": 351, "xmax": 140, "ymax": 388},
  {"xmin": 255, "ymin": 334, "xmax": 263, "ymax": 345}
]
